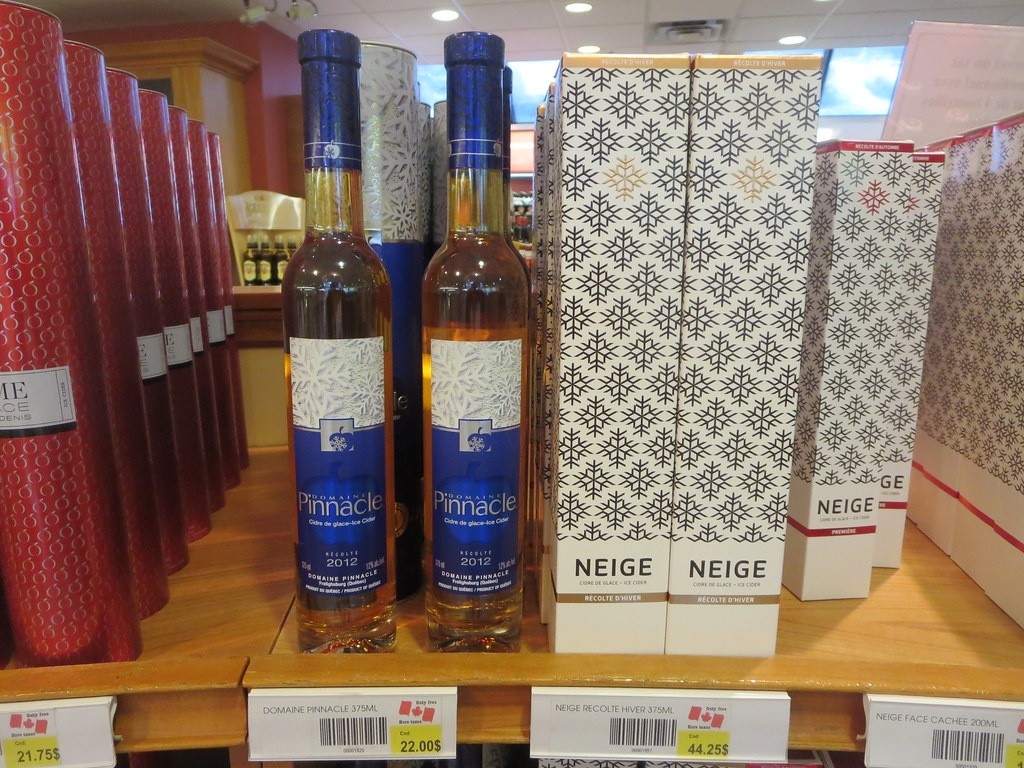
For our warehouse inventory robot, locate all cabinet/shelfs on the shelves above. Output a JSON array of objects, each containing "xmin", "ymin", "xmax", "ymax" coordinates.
[
  {"xmin": 82, "ymin": 36, "xmax": 262, "ymax": 197},
  {"xmin": 0, "ymin": 285, "xmax": 297, "ymax": 768},
  {"xmin": 237, "ymin": 285, "xmax": 1023, "ymax": 767}
]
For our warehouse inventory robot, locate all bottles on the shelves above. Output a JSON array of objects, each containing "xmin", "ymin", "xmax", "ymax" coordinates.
[{"xmin": 243, "ymin": 30, "xmax": 534, "ymax": 655}]
